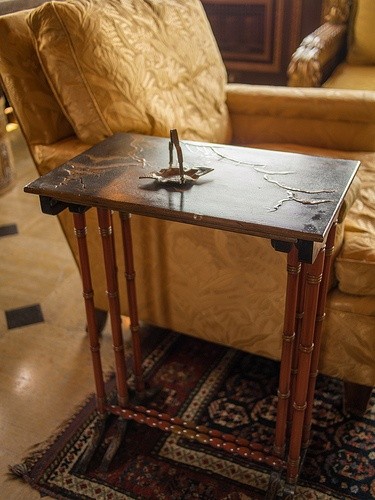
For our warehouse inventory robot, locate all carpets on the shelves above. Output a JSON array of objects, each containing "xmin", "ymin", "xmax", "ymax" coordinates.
[{"xmin": 4, "ymin": 323, "xmax": 375, "ymax": 500}]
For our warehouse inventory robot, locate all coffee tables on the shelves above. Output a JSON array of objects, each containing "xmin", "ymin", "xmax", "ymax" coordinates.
[{"xmin": 23, "ymin": 130, "xmax": 362, "ymax": 495}]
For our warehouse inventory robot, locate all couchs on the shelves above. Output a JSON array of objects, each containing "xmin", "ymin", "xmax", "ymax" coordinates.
[{"xmin": 1, "ymin": 0, "xmax": 375, "ymax": 389}]
